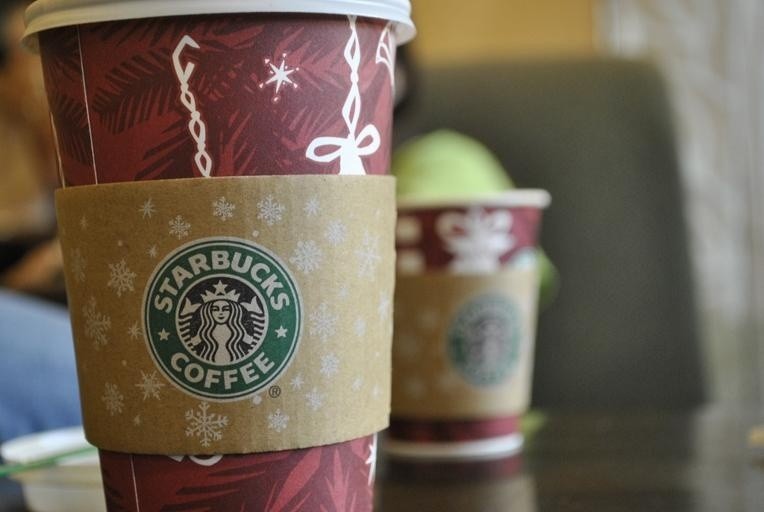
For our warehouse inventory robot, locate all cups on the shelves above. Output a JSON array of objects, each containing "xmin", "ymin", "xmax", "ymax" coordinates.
[
  {"xmin": 21, "ymin": 1, "xmax": 418, "ymax": 512},
  {"xmin": 380, "ymin": 188, "xmax": 553, "ymax": 462}
]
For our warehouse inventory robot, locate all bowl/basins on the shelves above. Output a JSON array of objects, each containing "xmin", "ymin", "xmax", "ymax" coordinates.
[{"xmin": 0, "ymin": 425, "xmax": 108, "ymax": 512}]
